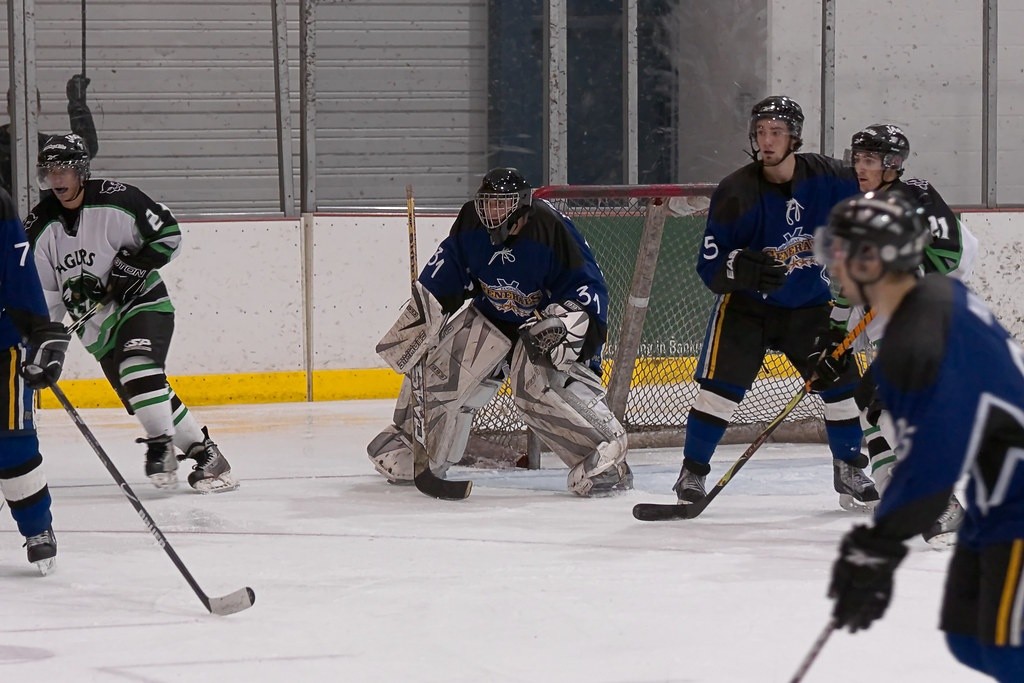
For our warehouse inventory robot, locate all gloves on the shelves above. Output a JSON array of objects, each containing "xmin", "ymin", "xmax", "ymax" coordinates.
[
  {"xmin": 725, "ymin": 248, "xmax": 789, "ymax": 294},
  {"xmin": 105, "ymin": 245, "xmax": 154, "ymax": 307},
  {"xmin": 21, "ymin": 322, "xmax": 71, "ymax": 390},
  {"xmin": 828, "ymin": 525, "xmax": 909, "ymax": 635},
  {"xmin": 806, "ymin": 328, "xmax": 853, "ymax": 394}
]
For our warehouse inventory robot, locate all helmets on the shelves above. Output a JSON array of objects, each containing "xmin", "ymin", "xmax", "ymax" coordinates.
[
  {"xmin": 750, "ymin": 96, "xmax": 804, "ymax": 141},
  {"xmin": 827, "ymin": 191, "xmax": 928, "ymax": 274},
  {"xmin": 38, "ymin": 132, "xmax": 91, "ymax": 177},
  {"xmin": 851, "ymin": 123, "xmax": 910, "ymax": 161},
  {"xmin": 474, "ymin": 167, "xmax": 532, "ymax": 244}
]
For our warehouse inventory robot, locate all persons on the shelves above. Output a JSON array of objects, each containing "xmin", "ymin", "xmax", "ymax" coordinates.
[
  {"xmin": 0, "ymin": 187, "xmax": 72, "ymax": 563},
  {"xmin": 826, "ymin": 191, "xmax": 1024, "ymax": 683},
  {"xmin": 22, "ymin": 133, "xmax": 232, "ymax": 489},
  {"xmin": 672, "ymin": 96, "xmax": 881, "ymax": 505},
  {"xmin": 803, "ymin": 123, "xmax": 978, "ymax": 544},
  {"xmin": 0, "ymin": 74, "xmax": 98, "ymax": 200},
  {"xmin": 366, "ymin": 166, "xmax": 629, "ymax": 498}
]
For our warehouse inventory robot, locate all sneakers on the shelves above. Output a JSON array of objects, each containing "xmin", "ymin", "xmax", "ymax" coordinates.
[
  {"xmin": 585, "ymin": 458, "xmax": 634, "ymax": 497},
  {"xmin": 921, "ymin": 493, "xmax": 967, "ymax": 551},
  {"xmin": 673, "ymin": 458, "xmax": 712, "ymax": 505},
  {"xmin": 135, "ymin": 434, "xmax": 179, "ymax": 488},
  {"xmin": 833, "ymin": 460, "xmax": 881, "ymax": 517},
  {"xmin": 176, "ymin": 426, "xmax": 240, "ymax": 494},
  {"xmin": 387, "ymin": 472, "xmax": 447, "ymax": 486},
  {"xmin": 26, "ymin": 526, "xmax": 56, "ymax": 576}
]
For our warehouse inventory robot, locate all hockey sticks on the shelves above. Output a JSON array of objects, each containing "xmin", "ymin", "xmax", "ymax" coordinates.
[
  {"xmin": 406, "ymin": 184, "xmax": 475, "ymax": 501},
  {"xmin": 67, "ymin": 300, "xmax": 109, "ymax": 337},
  {"xmin": 632, "ymin": 308, "xmax": 876, "ymax": 522},
  {"xmin": 791, "ymin": 622, "xmax": 836, "ymax": 682},
  {"xmin": 52, "ymin": 385, "xmax": 256, "ymax": 617}
]
